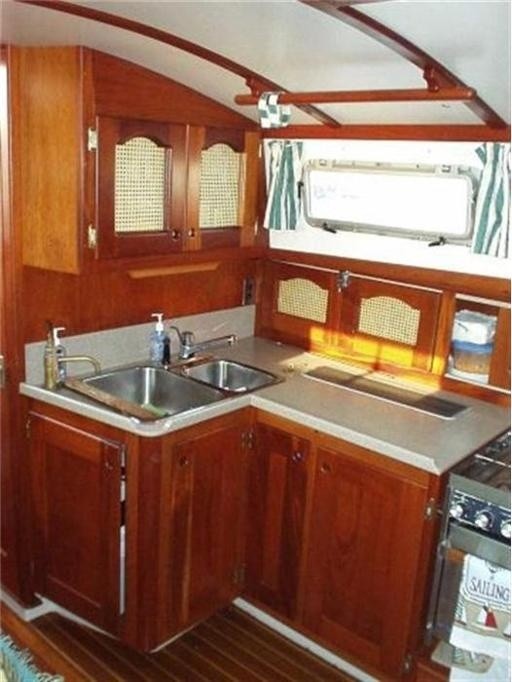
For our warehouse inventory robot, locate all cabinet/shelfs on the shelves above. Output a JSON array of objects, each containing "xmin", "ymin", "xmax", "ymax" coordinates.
[{"xmin": 1, "ymin": 46, "xmax": 443, "ymax": 677}]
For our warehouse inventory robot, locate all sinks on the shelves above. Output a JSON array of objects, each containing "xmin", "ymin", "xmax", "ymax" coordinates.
[
  {"xmin": 78, "ymin": 363, "xmax": 231, "ymax": 419},
  {"xmin": 168, "ymin": 356, "xmax": 286, "ymax": 394}
]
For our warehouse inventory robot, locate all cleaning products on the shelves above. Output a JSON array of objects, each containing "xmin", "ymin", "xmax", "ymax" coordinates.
[
  {"xmin": 49, "ymin": 327, "xmax": 67, "ymax": 378},
  {"xmin": 149, "ymin": 312, "xmax": 171, "ymax": 363}
]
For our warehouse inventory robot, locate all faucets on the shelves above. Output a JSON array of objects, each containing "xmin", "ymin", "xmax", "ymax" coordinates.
[
  {"xmin": 169, "ymin": 326, "xmax": 238, "ymax": 358},
  {"xmin": 44, "ymin": 331, "xmax": 99, "ymax": 391}
]
